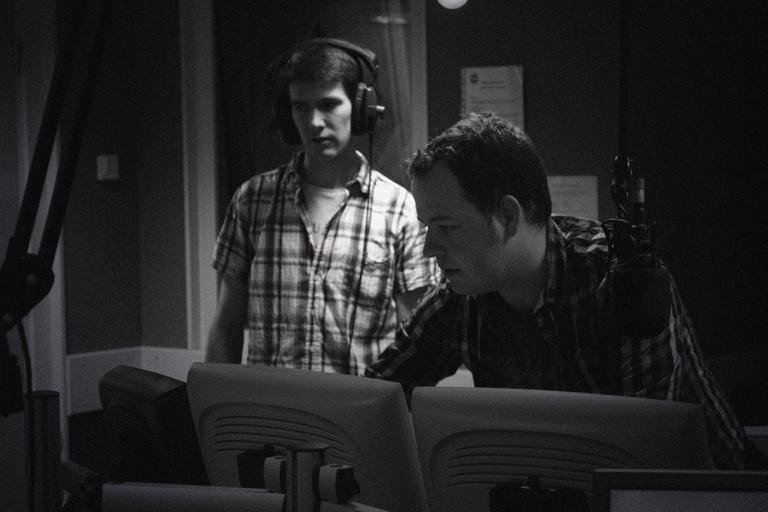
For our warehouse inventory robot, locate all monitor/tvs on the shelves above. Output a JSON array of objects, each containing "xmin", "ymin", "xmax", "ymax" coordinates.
[
  {"xmin": 97, "ymin": 363, "xmax": 209, "ymax": 486},
  {"xmin": 187, "ymin": 360, "xmax": 430, "ymax": 512},
  {"xmin": 591, "ymin": 469, "xmax": 768, "ymax": 512},
  {"xmin": 411, "ymin": 386, "xmax": 709, "ymax": 512}
]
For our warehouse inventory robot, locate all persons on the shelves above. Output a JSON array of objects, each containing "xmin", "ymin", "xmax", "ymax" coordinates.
[
  {"xmin": 364, "ymin": 110, "xmax": 768, "ymax": 471},
  {"xmin": 205, "ymin": 37, "xmax": 446, "ymax": 377}
]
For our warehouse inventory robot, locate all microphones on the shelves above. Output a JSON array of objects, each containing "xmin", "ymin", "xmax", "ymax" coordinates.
[{"xmin": 596, "ymin": 173, "xmax": 673, "ymax": 342}]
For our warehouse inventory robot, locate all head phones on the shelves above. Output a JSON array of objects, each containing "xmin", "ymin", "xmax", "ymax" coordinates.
[{"xmin": 266, "ymin": 37, "xmax": 386, "ymax": 146}]
